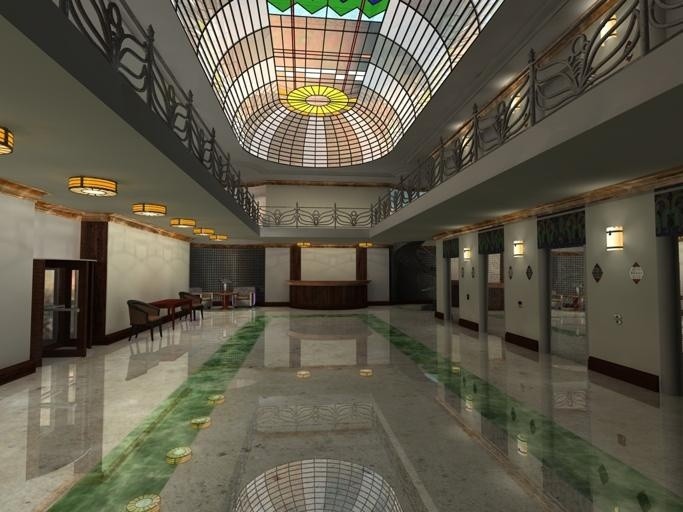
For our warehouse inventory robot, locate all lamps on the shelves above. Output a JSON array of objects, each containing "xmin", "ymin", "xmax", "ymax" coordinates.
[
  {"xmin": 170, "ymin": 218, "xmax": 229, "ymax": 242},
  {"xmin": 463, "ymin": 248, "xmax": 470, "ymax": 262},
  {"xmin": 131, "ymin": 203, "xmax": 167, "ymax": 216},
  {"xmin": 513, "ymin": 240, "xmax": 524, "ymax": 258},
  {"xmin": 0, "ymin": 127, "xmax": 14, "ymax": 155},
  {"xmin": 296, "ymin": 242, "xmax": 312, "ymax": 249},
  {"xmin": 359, "ymin": 242, "xmax": 373, "ymax": 248},
  {"xmin": 606, "ymin": 226, "xmax": 625, "ymax": 251},
  {"xmin": 68, "ymin": 176, "xmax": 118, "ymax": 197},
  {"xmin": 599, "ymin": 13, "xmax": 617, "ymax": 46},
  {"xmin": 510, "ymin": 92, "xmax": 520, "ymax": 112}
]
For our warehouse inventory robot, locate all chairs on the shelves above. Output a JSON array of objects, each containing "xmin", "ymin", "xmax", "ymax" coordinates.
[
  {"xmin": 125, "ymin": 320, "xmax": 200, "ymax": 382},
  {"xmin": 127, "ymin": 286, "xmax": 257, "ymax": 341}
]
